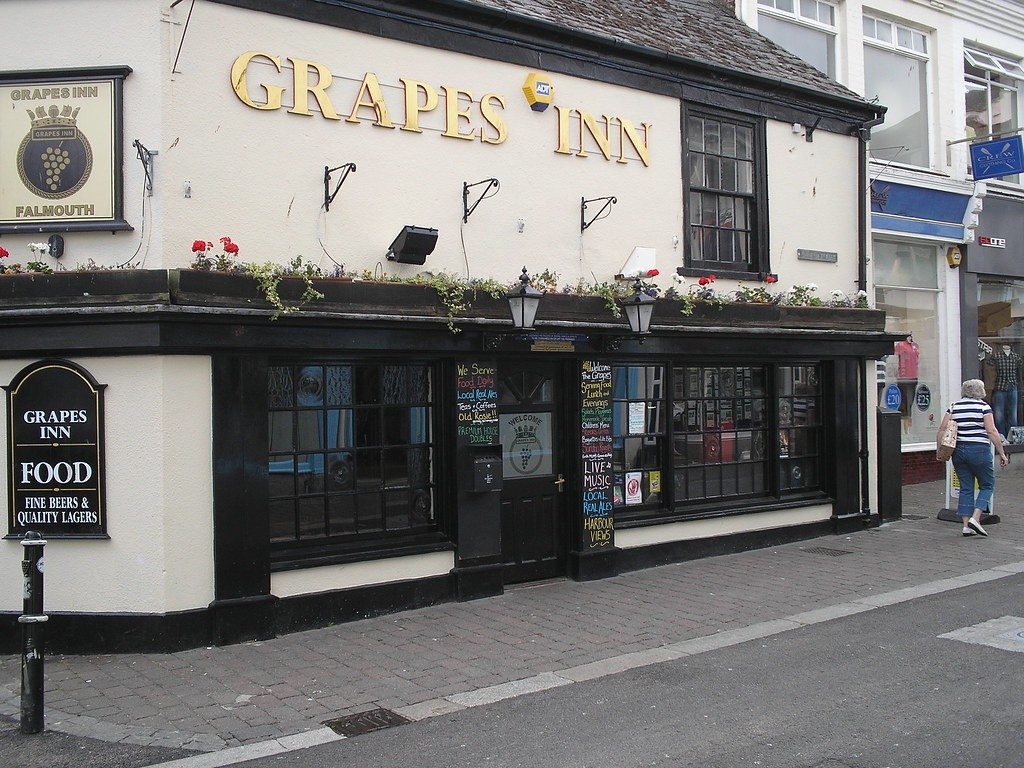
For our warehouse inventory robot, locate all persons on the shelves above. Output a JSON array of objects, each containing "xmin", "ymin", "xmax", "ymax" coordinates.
[
  {"xmin": 794, "ymin": 382, "xmax": 815, "ymax": 426},
  {"xmin": 990, "ymin": 344, "xmax": 1024, "ymax": 444},
  {"xmin": 895, "ymin": 335, "xmax": 920, "ymax": 435},
  {"xmin": 935, "ymin": 380, "xmax": 1006, "ymax": 537}
]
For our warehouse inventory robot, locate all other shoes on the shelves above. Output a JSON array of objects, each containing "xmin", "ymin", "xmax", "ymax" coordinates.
[
  {"xmin": 968, "ymin": 517, "xmax": 988, "ymax": 536},
  {"xmin": 962, "ymin": 526, "xmax": 977, "ymax": 536}
]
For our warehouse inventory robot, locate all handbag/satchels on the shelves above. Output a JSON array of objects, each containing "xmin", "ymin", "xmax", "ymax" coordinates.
[{"xmin": 936, "ymin": 403, "xmax": 957, "ymax": 461}]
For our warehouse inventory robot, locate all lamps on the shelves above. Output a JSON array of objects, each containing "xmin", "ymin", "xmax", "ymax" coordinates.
[
  {"xmin": 505, "ymin": 265, "xmax": 543, "ymax": 332},
  {"xmin": 620, "ymin": 275, "xmax": 656, "ymax": 344},
  {"xmin": 385, "ymin": 225, "xmax": 438, "ymax": 265}
]
